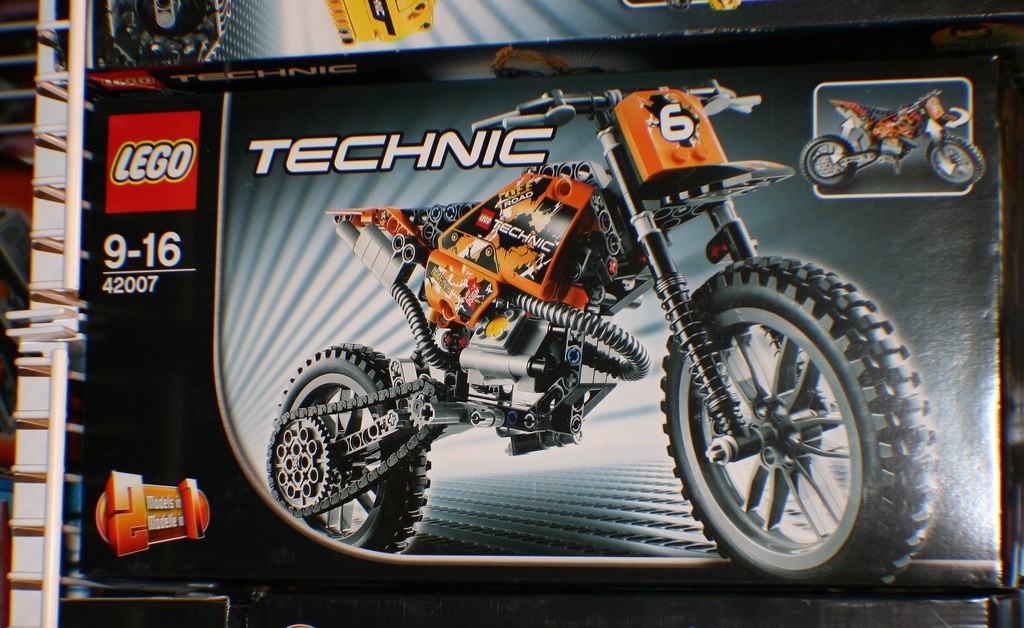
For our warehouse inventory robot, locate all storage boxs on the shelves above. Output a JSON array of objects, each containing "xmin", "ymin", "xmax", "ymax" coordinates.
[
  {"xmin": 87, "ymin": 0, "xmax": 1024, "ymax": 94},
  {"xmin": 79, "ymin": 50, "xmax": 1007, "ymax": 590}
]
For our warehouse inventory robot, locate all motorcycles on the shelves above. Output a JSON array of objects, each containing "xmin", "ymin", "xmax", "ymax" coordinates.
[
  {"xmin": 263, "ymin": 80, "xmax": 943, "ymax": 601},
  {"xmin": 800, "ymin": 81, "xmax": 984, "ymax": 192}
]
